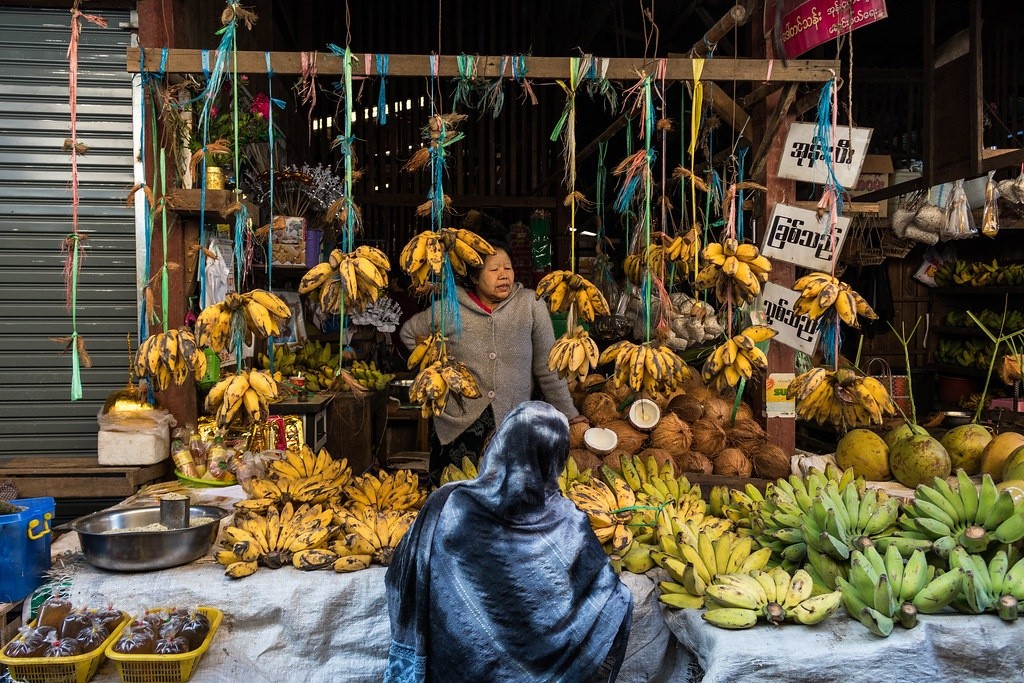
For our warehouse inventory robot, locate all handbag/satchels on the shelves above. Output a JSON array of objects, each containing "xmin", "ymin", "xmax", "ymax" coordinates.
[{"xmin": 862, "ymin": 357, "xmax": 912, "ymax": 418}]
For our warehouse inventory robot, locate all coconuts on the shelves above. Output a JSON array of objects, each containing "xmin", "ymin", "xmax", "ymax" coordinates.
[
  {"xmin": 836, "ymin": 422, "xmax": 1024, "ymax": 509},
  {"xmin": 563, "ymin": 365, "xmax": 790, "ymax": 480}
]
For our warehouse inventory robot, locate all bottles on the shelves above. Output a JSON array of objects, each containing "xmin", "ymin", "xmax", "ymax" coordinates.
[{"xmin": 170, "ymin": 434, "xmax": 227, "ymax": 482}]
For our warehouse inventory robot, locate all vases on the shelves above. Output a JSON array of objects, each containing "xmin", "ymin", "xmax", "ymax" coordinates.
[{"xmin": 206, "ymin": 166, "xmax": 226, "ymax": 191}]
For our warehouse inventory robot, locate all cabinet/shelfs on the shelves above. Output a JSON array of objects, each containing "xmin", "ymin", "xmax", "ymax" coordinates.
[
  {"xmin": 927, "ymin": 218, "xmax": 1024, "ymax": 379},
  {"xmin": 267, "ymin": 386, "xmax": 375, "ymax": 470}
]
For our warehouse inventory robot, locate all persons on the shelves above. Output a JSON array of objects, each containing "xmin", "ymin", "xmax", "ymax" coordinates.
[
  {"xmin": 384, "ymin": 401, "xmax": 632, "ymax": 683},
  {"xmin": 401, "ymin": 243, "xmax": 587, "ymax": 487}
]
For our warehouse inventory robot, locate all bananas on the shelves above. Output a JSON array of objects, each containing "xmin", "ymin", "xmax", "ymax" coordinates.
[
  {"xmin": 555, "ymin": 452, "xmax": 1024, "ymax": 638},
  {"xmin": 135, "ymin": 227, "xmax": 498, "ymax": 577},
  {"xmin": 536, "ymin": 231, "xmax": 896, "ymax": 428},
  {"xmin": 936, "ymin": 258, "xmax": 1024, "ymax": 413}
]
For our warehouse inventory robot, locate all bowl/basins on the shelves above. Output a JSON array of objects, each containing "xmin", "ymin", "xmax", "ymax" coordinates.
[
  {"xmin": 388, "ymin": 379, "xmax": 414, "ymax": 402},
  {"xmin": 67, "ymin": 504, "xmax": 230, "ymax": 572},
  {"xmin": 940, "ymin": 410, "xmax": 975, "ymax": 428}
]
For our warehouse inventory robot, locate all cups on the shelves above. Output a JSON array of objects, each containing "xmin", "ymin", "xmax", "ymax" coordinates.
[
  {"xmin": 290, "ymin": 376, "xmax": 305, "ymax": 394},
  {"xmin": 160, "ymin": 497, "xmax": 190, "ymax": 529}
]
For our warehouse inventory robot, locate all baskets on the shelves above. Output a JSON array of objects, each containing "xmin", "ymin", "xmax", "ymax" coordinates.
[
  {"xmin": 105, "ymin": 605, "xmax": 224, "ymax": 683},
  {"xmin": 0, "ymin": 608, "xmax": 130, "ymax": 683}
]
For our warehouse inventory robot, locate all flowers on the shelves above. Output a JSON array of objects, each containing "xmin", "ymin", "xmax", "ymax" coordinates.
[{"xmin": 161, "ymin": 71, "xmax": 281, "ymax": 169}]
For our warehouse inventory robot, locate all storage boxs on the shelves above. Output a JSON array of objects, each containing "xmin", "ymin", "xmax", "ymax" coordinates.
[
  {"xmin": 97, "ymin": 431, "xmax": 171, "ymax": 465},
  {"xmin": 271, "ymin": 215, "xmax": 307, "ymax": 266},
  {"xmin": 0, "ymin": 496, "xmax": 56, "ymax": 604},
  {"xmin": 843, "ymin": 154, "xmax": 893, "ymax": 216}
]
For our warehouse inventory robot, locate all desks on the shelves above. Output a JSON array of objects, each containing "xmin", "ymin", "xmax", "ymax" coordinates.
[
  {"xmin": 386, "ymin": 398, "xmax": 430, "ymax": 482},
  {"xmin": 0, "ymin": 457, "xmax": 170, "ymax": 500}
]
